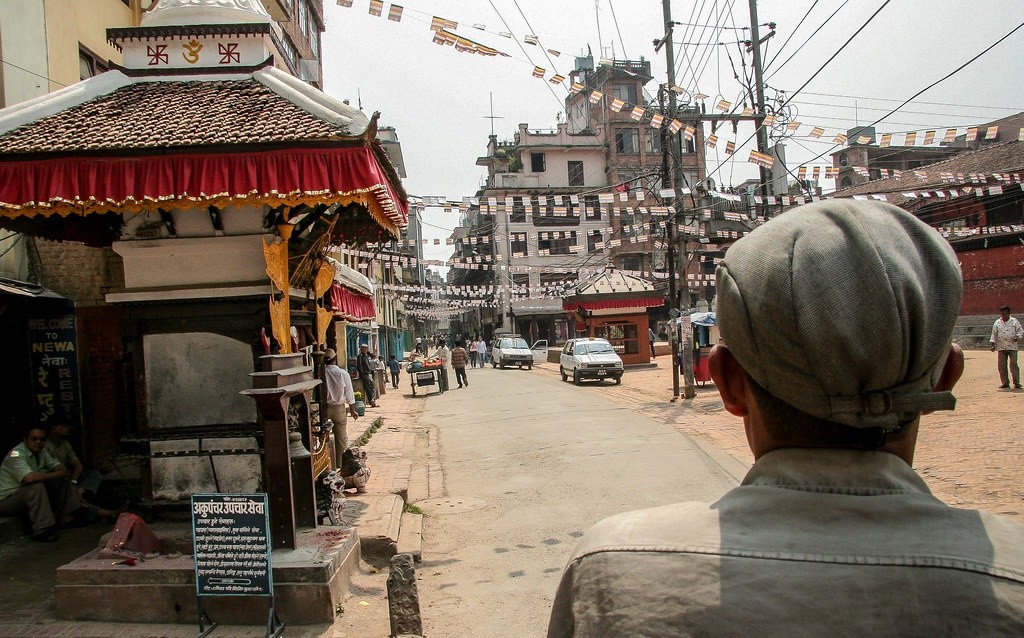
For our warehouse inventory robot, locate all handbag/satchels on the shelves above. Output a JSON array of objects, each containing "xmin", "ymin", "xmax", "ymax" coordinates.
[{"xmin": 485, "ymin": 354, "xmax": 488, "ymax": 363}]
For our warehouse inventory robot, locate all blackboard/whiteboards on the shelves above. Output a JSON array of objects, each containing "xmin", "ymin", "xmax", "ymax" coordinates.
[{"xmin": 190, "ymin": 492, "xmax": 274, "ymax": 596}]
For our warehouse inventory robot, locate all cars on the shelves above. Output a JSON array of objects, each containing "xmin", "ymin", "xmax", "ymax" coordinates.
[
  {"xmin": 560, "ymin": 337, "xmax": 625, "ymax": 387},
  {"xmin": 492, "ymin": 334, "xmax": 548, "ymax": 369}
]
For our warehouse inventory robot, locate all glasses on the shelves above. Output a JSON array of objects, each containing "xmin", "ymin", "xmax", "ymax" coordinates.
[{"xmin": 31, "ymin": 437, "xmax": 47, "ymax": 442}]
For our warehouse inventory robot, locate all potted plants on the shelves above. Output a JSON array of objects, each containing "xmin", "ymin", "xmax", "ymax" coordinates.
[{"xmin": 354, "ymin": 391, "xmax": 365, "ymax": 415}]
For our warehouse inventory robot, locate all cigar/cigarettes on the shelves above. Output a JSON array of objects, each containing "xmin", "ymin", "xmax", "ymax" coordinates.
[{"xmin": 354, "ymin": 419, "xmax": 356, "ymax": 423}]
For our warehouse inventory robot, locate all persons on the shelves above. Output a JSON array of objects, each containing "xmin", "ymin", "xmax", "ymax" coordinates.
[
  {"xmin": 0, "ymin": 425, "xmax": 74, "ymax": 541},
  {"xmin": 390, "ymin": 354, "xmax": 400, "ymax": 389},
  {"xmin": 415, "ymin": 332, "xmax": 487, "ymax": 391},
  {"xmin": 546, "ymin": 196, "xmax": 1024, "ymax": 638},
  {"xmin": 324, "ymin": 348, "xmax": 358, "ymax": 467},
  {"xmin": 38, "ymin": 412, "xmax": 131, "ymax": 523},
  {"xmin": 357, "ymin": 343, "xmax": 390, "ymax": 408}
]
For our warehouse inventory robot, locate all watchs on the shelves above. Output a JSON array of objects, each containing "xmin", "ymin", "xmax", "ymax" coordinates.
[{"xmin": 69, "ymin": 480, "xmax": 78, "ymax": 485}]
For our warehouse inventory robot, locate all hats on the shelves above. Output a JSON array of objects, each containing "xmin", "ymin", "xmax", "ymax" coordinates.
[
  {"xmin": 714, "ymin": 198, "xmax": 963, "ymax": 434},
  {"xmin": 324, "ymin": 348, "xmax": 336, "ymax": 361},
  {"xmin": 361, "ymin": 343, "xmax": 369, "ymax": 348}
]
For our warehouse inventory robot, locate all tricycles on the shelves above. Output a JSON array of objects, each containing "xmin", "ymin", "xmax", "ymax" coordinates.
[{"xmin": 409, "ymin": 354, "xmax": 444, "ymax": 396}]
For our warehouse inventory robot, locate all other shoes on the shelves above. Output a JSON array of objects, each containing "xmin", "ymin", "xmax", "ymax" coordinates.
[
  {"xmin": 998, "ymin": 385, "xmax": 1009, "ymax": 388},
  {"xmin": 464, "ymin": 380, "xmax": 468, "ymax": 386},
  {"xmin": 1015, "ymin": 384, "xmax": 1021, "ymax": 388},
  {"xmin": 372, "ymin": 404, "xmax": 380, "ymax": 407},
  {"xmin": 458, "ymin": 384, "xmax": 462, "ymax": 388}
]
